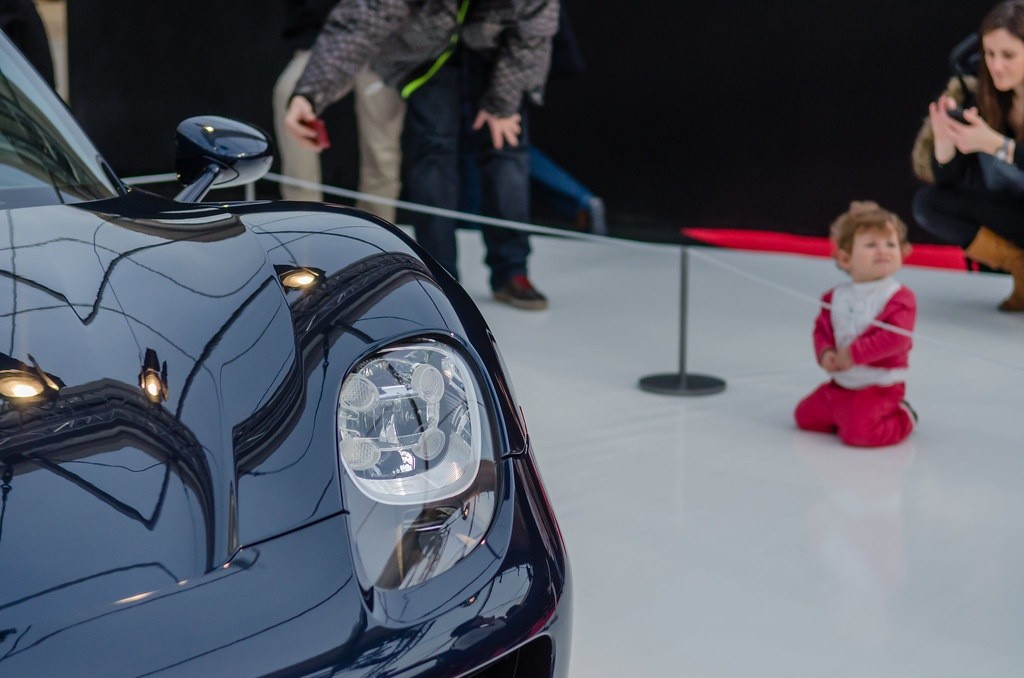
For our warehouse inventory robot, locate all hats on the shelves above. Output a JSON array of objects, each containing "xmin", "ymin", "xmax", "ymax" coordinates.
[{"xmin": 833, "ymin": 201, "xmax": 906, "ymax": 245}]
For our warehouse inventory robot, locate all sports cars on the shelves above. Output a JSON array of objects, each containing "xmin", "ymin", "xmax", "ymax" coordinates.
[{"xmin": 0, "ymin": 25, "xmax": 575, "ymax": 678}]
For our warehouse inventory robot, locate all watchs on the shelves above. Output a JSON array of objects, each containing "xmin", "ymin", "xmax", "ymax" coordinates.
[{"xmin": 996, "ymin": 138, "xmax": 1010, "ymax": 161}]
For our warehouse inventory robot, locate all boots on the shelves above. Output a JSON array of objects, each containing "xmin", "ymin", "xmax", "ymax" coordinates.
[{"xmin": 964, "ymin": 226, "xmax": 1024, "ymax": 311}]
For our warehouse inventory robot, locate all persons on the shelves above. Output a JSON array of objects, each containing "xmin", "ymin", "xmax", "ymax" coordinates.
[
  {"xmin": 794, "ymin": 201, "xmax": 918, "ymax": 448},
  {"xmin": 272, "ymin": 0, "xmax": 407, "ymax": 227},
  {"xmin": 283, "ymin": 0, "xmax": 558, "ymax": 310},
  {"xmin": 912, "ymin": 0, "xmax": 1024, "ymax": 312}
]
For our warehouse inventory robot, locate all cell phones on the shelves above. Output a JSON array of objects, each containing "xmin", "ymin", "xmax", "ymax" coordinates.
[
  {"xmin": 947, "ymin": 107, "xmax": 972, "ymax": 126},
  {"xmin": 299, "ymin": 120, "xmax": 329, "ymax": 150}
]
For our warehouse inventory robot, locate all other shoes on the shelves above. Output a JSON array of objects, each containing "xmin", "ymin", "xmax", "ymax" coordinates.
[{"xmin": 494, "ymin": 273, "xmax": 548, "ymax": 309}]
